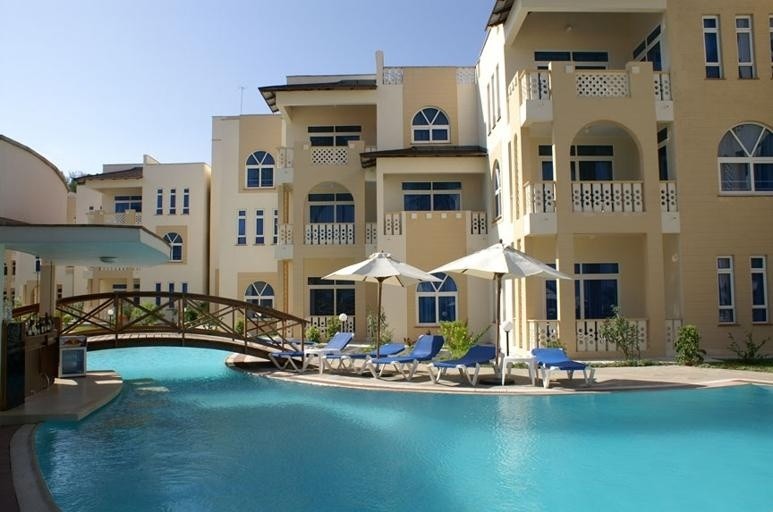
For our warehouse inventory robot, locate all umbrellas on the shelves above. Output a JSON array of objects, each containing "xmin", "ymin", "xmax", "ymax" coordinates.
[
  {"xmin": 426, "ymin": 239, "xmax": 575, "ymax": 366},
  {"xmin": 319, "ymin": 249, "xmax": 443, "ymax": 359}
]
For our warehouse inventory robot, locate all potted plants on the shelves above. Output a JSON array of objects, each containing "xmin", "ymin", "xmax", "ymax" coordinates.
[{"xmin": 673, "ymin": 326, "xmax": 707, "ymax": 366}]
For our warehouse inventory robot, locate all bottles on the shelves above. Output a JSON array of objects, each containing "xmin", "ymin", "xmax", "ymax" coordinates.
[{"xmin": 27, "ymin": 313, "xmax": 52, "ymax": 336}]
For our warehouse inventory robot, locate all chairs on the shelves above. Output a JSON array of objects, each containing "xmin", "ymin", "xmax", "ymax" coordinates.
[
  {"xmin": 367, "ymin": 335, "xmax": 444, "ymax": 382},
  {"xmin": 532, "ymin": 347, "xmax": 594, "ymax": 388},
  {"xmin": 268, "ymin": 332, "xmax": 354, "ymax": 374},
  {"xmin": 319, "ymin": 342, "xmax": 410, "ymax": 375},
  {"xmin": 433, "ymin": 345, "xmax": 499, "ymax": 388}
]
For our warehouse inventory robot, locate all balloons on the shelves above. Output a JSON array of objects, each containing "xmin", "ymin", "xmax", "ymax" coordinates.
[{"xmin": 339, "ymin": 313, "xmax": 346, "ymax": 321}]
